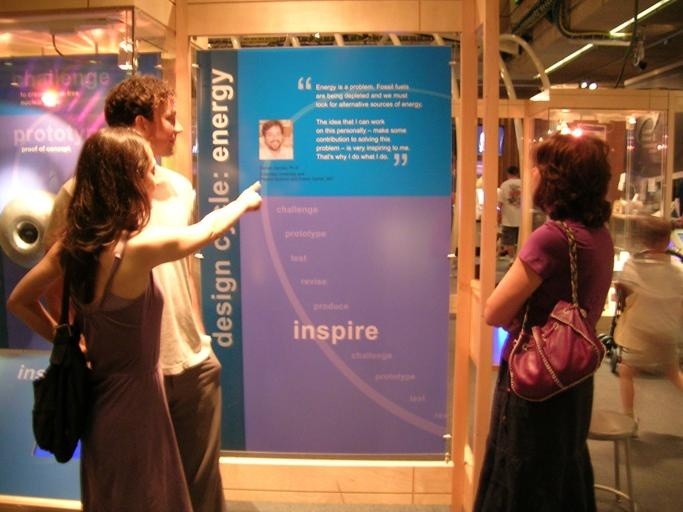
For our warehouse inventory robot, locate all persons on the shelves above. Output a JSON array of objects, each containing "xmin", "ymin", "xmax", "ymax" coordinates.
[
  {"xmin": 42, "ymin": 75, "xmax": 226, "ymax": 510},
  {"xmin": 259, "ymin": 120, "xmax": 293, "ymax": 159},
  {"xmin": 611, "ymin": 217, "xmax": 683, "ymax": 439},
  {"xmin": 6, "ymin": 127, "xmax": 263, "ymax": 511},
  {"xmin": 497, "ymin": 164, "xmax": 523, "ymax": 268},
  {"xmin": 469, "ymin": 132, "xmax": 616, "ymax": 512}
]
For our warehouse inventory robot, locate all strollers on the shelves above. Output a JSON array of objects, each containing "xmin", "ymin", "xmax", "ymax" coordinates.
[{"xmin": 594, "ymin": 250, "xmax": 682, "ymax": 372}]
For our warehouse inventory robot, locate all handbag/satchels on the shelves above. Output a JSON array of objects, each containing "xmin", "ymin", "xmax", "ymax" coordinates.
[
  {"xmin": 33, "ymin": 324, "xmax": 102, "ymax": 463},
  {"xmin": 509, "ymin": 301, "xmax": 604, "ymax": 401}
]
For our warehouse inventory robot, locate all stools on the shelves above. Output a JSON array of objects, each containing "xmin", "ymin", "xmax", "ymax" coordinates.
[{"xmin": 585, "ymin": 408, "xmax": 640, "ymax": 512}]
